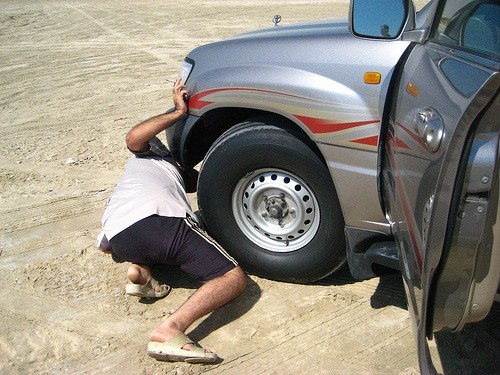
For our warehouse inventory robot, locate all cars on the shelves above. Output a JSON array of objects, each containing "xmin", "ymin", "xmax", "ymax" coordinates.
[{"xmin": 165, "ymin": 0, "xmax": 500, "ymax": 375}]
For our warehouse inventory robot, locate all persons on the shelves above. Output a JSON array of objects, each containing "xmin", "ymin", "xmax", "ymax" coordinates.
[{"xmin": 97, "ymin": 78, "xmax": 247, "ymax": 363}]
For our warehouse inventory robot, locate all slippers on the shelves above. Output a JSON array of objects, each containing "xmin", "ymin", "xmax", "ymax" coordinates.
[
  {"xmin": 147, "ymin": 332, "xmax": 217, "ymax": 363},
  {"xmin": 126, "ymin": 276, "xmax": 171, "ymax": 298}
]
